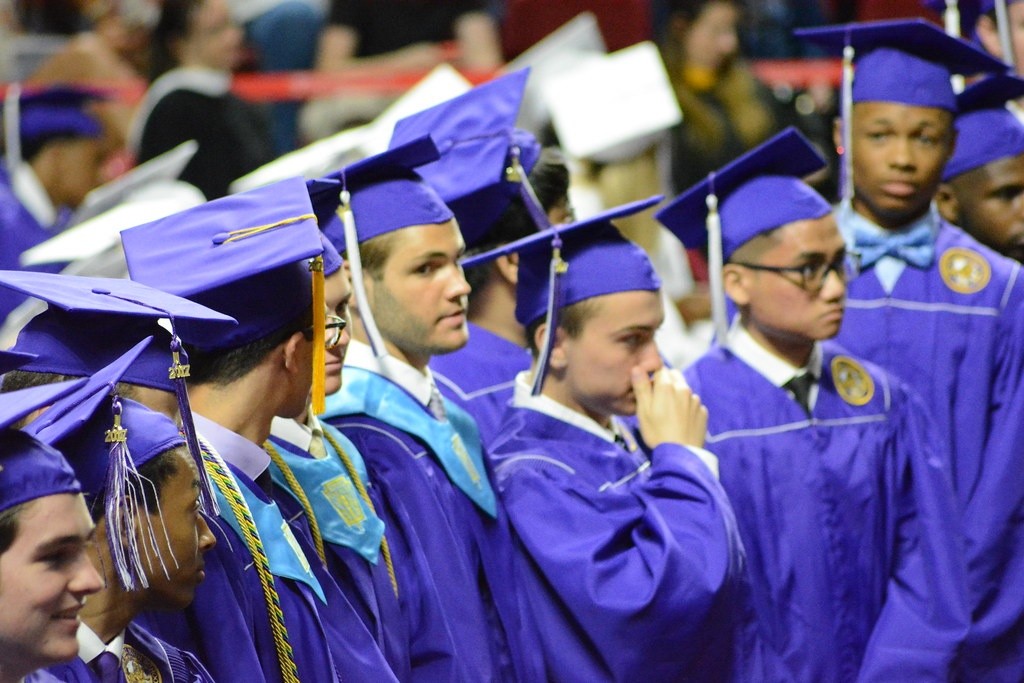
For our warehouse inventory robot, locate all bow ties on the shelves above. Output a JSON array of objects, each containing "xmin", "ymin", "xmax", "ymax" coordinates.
[{"xmin": 849, "ymin": 226, "xmax": 935, "ymax": 270}]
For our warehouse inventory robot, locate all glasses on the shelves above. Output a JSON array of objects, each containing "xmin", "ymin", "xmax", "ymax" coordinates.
[
  {"xmin": 276, "ymin": 314, "xmax": 346, "ymax": 353},
  {"xmin": 731, "ymin": 256, "xmax": 860, "ymax": 289}
]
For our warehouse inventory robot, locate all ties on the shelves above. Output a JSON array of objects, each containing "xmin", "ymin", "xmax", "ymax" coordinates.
[
  {"xmin": 782, "ymin": 374, "xmax": 815, "ymax": 418},
  {"xmin": 429, "ymin": 383, "xmax": 447, "ymax": 421}
]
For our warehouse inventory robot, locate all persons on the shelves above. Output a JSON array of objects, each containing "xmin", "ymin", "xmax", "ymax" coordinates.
[
  {"xmin": 0, "ymin": 0, "xmax": 284, "ymax": 332},
  {"xmin": 291, "ymin": 0, "xmax": 508, "ymax": 143},
  {"xmin": 463, "ymin": 125, "xmax": 970, "ymax": 680},
  {"xmin": 792, "ymin": 0, "xmax": 1024, "ymax": 683},
  {"xmin": 646, "ymin": 0, "xmax": 830, "ymax": 199},
  {"xmin": 0, "ymin": 133, "xmax": 548, "ymax": 683}
]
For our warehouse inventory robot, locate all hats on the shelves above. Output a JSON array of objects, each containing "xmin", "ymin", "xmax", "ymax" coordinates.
[
  {"xmin": 790, "ymin": 15, "xmax": 1014, "ymax": 199},
  {"xmin": 460, "ymin": 194, "xmax": 667, "ymax": 398},
  {"xmin": 652, "ymin": 126, "xmax": 835, "ymax": 347},
  {"xmin": 1, "ymin": 9, "xmax": 686, "ymax": 593},
  {"xmin": 939, "ymin": 71, "xmax": 1024, "ymax": 187}
]
